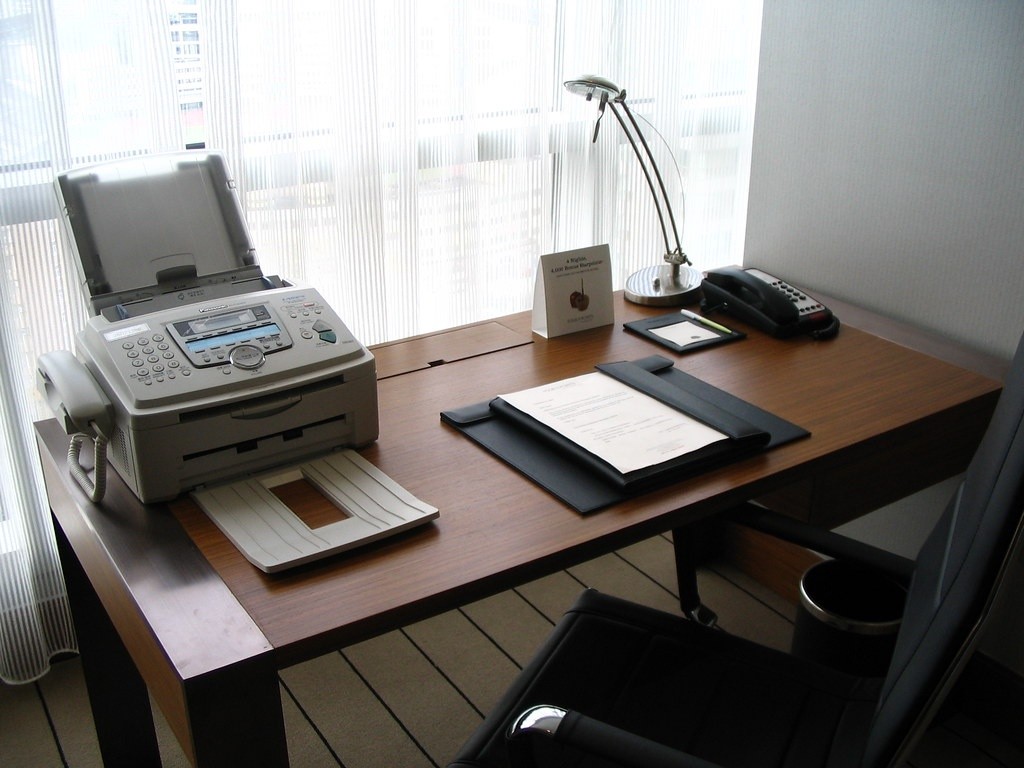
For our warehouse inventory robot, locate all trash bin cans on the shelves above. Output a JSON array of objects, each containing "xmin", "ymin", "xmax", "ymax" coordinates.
[{"xmin": 789, "ymin": 560, "xmax": 908, "ymax": 685}]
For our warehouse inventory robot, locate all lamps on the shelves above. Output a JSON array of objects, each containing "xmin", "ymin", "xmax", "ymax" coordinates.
[{"xmin": 562, "ymin": 72, "xmax": 703, "ymax": 306}]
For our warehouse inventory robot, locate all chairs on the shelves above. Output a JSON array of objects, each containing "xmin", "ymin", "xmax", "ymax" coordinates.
[{"xmin": 459, "ymin": 330, "xmax": 1024, "ymax": 768}]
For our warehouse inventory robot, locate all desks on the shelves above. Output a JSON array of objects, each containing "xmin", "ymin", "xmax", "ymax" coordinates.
[{"xmin": 28, "ymin": 288, "xmax": 1002, "ymax": 768}]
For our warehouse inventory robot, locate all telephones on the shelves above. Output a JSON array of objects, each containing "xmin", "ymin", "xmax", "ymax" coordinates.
[
  {"xmin": 701, "ymin": 265, "xmax": 840, "ymax": 342},
  {"xmin": 33, "ymin": 284, "xmax": 381, "ymax": 507}
]
